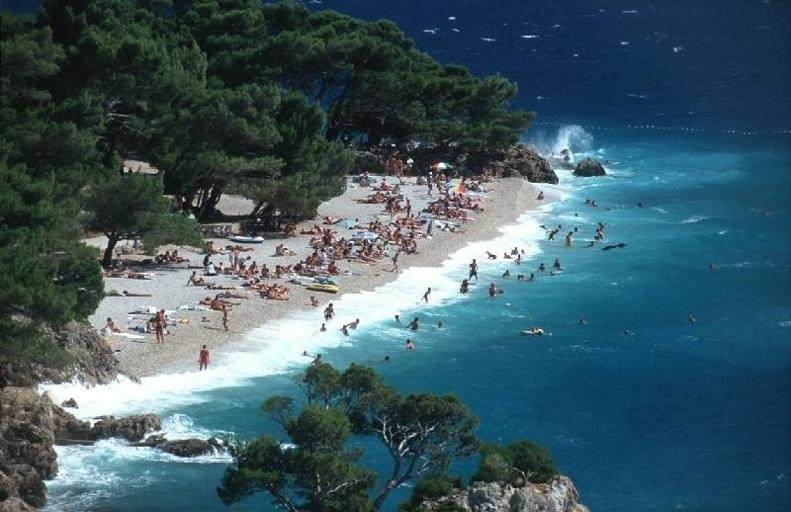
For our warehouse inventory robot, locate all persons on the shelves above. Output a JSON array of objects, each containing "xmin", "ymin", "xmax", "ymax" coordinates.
[{"xmin": 103, "ymin": 156, "xmax": 646, "ymax": 371}]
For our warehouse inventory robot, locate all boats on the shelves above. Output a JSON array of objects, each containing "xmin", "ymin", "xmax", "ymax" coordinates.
[
  {"xmin": 307, "ymin": 282, "xmax": 342, "ymax": 293},
  {"xmin": 230, "ymin": 234, "xmax": 265, "ymax": 244}
]
